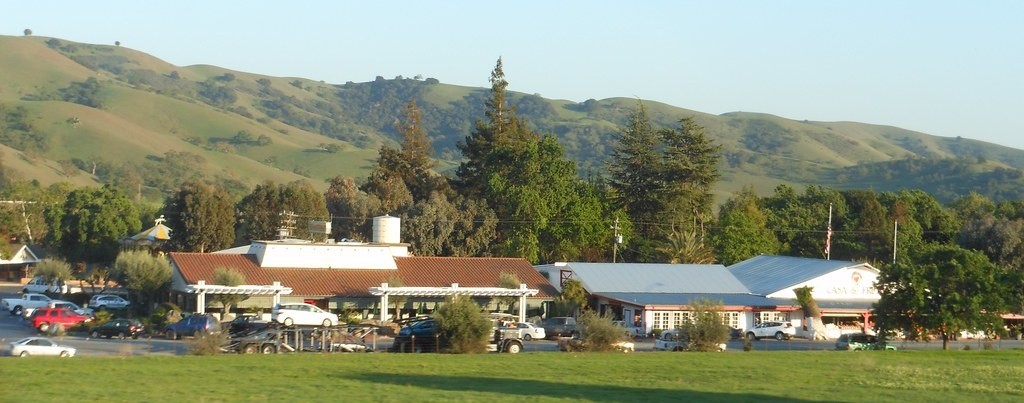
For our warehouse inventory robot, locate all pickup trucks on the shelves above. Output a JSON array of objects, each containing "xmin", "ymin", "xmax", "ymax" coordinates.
[
  {"xmin": 22, "ymin": 299, "xmax": 95, "ymax": 321},
  {"xmin": 1, "ymin": 293, "xmax": 52, "ymax": 316}
]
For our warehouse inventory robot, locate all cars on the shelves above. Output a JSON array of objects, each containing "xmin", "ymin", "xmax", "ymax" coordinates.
[
  {"xmin": 392, "ymin": 318, "xmax": 452, "ymax": 353},
  {"xmin": 22, "ymin": 275, "xmax": 67, "ymax": 294},
  {"xmin": 653, "ymin": 329, "xmax": 727, "ymax": 353},
  {"xmin": 488, "ymin": 313, "xmax": 520, "ymax": 344},
  {"xmin": 540, "ymin": 317, "xmax": 585, "ymax": 339},
  {"xmin": 88, "ymin": 294, "xmax": 131, "ymax": 311},
  {"xmin": 88, "ymin": 319, "xmax": 145, "ymax": 339},
  {"xmin": 743, "ymin": 320, "xmax": 796, "ymax": 340},
  {"xmin": 477, "ymin": 313, "xmax": 524, "ymax": 353},
  {"xmin": 271, "ymin": 302, "xmax": 339, "ymax": 327},
  {"xmin": 834, "ymin": 333, "xmax": 897, "ymax": 350},
  {"xmin": 557, "ymin": 335, "xmax": 635, "ymax": 353},
  {"xmin": 231, "ymin": 327, "xmax": 320, "ymax": 354},
  {"xmin": 955, "ymin": 322, "xmax": 996, "ymax": 341},
  {"xmin": 516, "ymin": 322, "xmax": 546, "ymax": 341},
  {"xmin": 610, "ymin": 321, "xmax": 638, "ymax": 340},
  {"xmin": 8, "ymin": 337, "xmax": 77, "ymax": 358},
  {"xmin": 722, "ymin": 325, "xmax": 743, "ymax": 342},
  {"xmin": 221, "ymin": 315, "xmax": 268, "ymax": 334},
  {"xmin": 162, "ymin": 313, "xmax": 222, "ymax": 340}
]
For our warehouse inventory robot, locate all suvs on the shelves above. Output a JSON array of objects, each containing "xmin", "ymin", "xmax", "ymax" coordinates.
[{"xmin": 30, "ymin": 307, "xmax": 96, "ymax": 332}]
